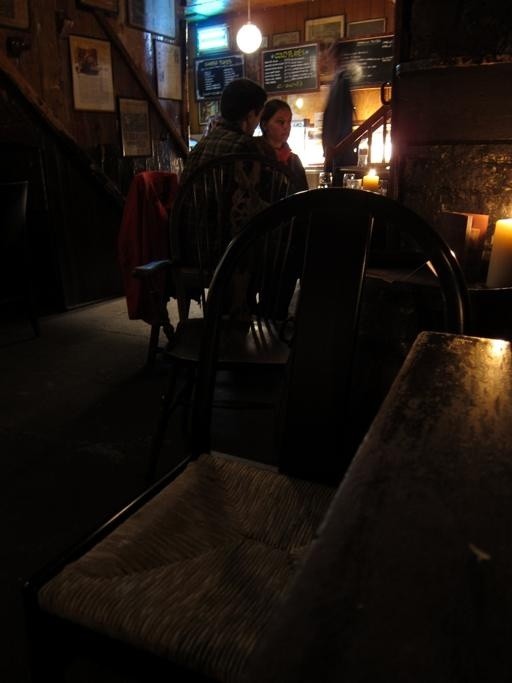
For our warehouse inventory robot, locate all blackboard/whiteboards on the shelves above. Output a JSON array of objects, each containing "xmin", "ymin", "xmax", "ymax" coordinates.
[{"xmin": 193, "ymin": 34, "xmax": 395, "ymax": 102}]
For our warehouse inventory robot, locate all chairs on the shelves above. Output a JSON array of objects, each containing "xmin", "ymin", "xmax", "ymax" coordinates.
[
  {"xmin": 192, "ymin": 188, "xmax": 468, "ymax": 456},
  {"xmin": 118, "ymin": 171, "xmax": 209, "ymax": 361}
]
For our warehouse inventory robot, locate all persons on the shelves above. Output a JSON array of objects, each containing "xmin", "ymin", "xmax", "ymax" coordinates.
[
  {"xmin": 169, "ymin": 74, "xmax": 297, "ymax": 275},
  {"xmin": 251, "ymin": 97, "xmax": 310, "ymax": 320},
  {"xmin": 318, "ymin": 38, "xmax": 360, "ymax": 190}
]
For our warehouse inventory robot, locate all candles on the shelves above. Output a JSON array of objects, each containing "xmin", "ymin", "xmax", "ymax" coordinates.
[{"xmin": 486, "ymin": 218, "xmax": 512, "ymax": 288}]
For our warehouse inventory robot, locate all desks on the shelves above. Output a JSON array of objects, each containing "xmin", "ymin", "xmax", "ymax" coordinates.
[{"xmin": 237, "ymin": 323, "xmax": 512, "ymax": 682}]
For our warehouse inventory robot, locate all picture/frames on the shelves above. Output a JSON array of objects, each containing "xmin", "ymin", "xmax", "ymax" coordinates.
[
  {"xmin": 0, "ymin": 0, "xmax": 37, "ymax": 34},
  {"xmin": 302, "ymin": 13, "xmax": 348, "ymax": 87},
  {"xmin": 65, "ymin": 29, "xmax": 153, "ymax": 161}
]
